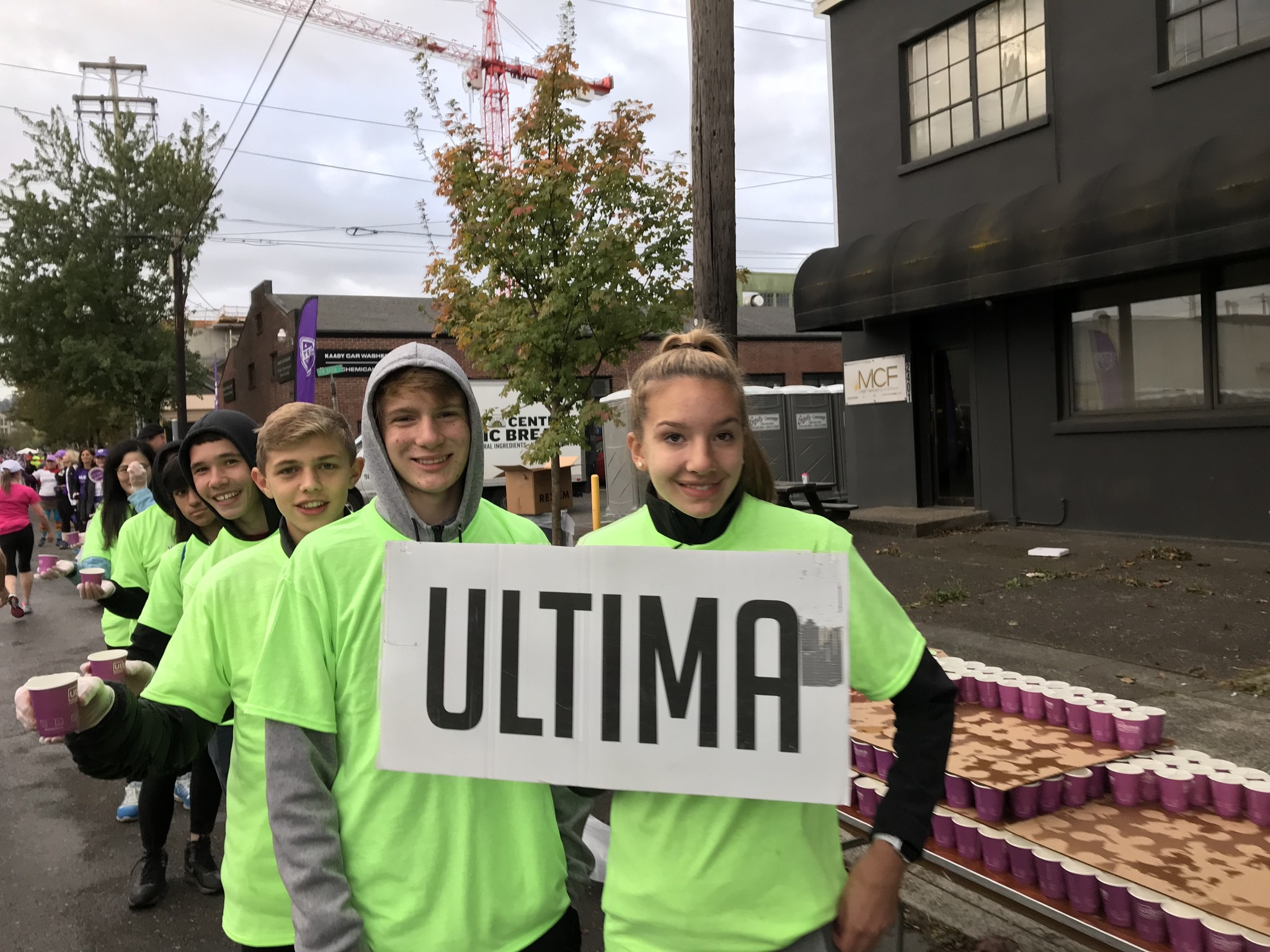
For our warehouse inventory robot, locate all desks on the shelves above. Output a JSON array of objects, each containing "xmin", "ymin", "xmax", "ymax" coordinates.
[{"xmin": 836, "ymin": 805, "xmax": 1171, "ymax": 952}]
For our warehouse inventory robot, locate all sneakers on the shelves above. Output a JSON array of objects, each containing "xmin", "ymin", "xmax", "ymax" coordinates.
[
  {"xmin": 129, "ymin": 848, "xmax": 168, "ymax": 910},
  {"xmin": 116, "ymin": 780, "xmax": 142, "ymax": 821},
  {"xmin": 174, "ymin": 772, "xmax": 192, "ymax": 809},
  {"xmin": 183, "ymin": 837, "xmax": 223, "ymax": 896},
  {"xmin": 8, "ymin": 594, "xmax": 24, "ymax": 618},
  {"xmin": 18, "ymin": 601, "xmax": 31, "ymax": 614}
]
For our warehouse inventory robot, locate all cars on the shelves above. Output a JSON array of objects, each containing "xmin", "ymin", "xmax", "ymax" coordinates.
[{"xmin": 592, "ymin": 436, "xmax": 606, "ymax": 486}]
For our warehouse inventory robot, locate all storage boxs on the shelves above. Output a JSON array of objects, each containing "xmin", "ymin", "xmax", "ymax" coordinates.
[{"xmin": 492, "ymin": 455, "xmax": 579, "ymax": 515}]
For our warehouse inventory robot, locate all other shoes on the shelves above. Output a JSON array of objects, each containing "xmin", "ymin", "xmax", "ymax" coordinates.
[
  {"xmin": 55, "ymin": 538, "xmax": 62, "ymax": 546},
  {"xmin": 59, "ymin": 541, "xmax": 68, "ymax": 549},
  {"xmin": 37, "ymin": 537, "xmax": 46, "ymax": 547}
]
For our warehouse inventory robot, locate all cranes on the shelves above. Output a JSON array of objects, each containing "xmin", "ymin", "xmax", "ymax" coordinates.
[{"xmin": 234, "ymin": 0, "xmax": 615, "ymax": 296}]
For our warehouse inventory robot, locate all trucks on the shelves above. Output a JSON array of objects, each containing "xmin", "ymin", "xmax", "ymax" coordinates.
[{"xmin": 346, "ymin": 378, "xmax": 590, "ymax": 511}]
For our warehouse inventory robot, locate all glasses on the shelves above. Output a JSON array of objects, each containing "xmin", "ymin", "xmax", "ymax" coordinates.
[{"xmin": 116, "ymin": 463, "xmax": 150, "ymax": 476}]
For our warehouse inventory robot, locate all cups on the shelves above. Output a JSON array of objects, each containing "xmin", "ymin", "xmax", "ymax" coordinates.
[
  {"xmin": 1151, "ymin": 745, "xmax": 1215, "ymax": 813},
  {"xmin": 1012, "ymin": 767, "xmax": 1094, "ymax": 819},
  {"xmin": 38, "ymin": 554, "xmax": 59, "ymax": 575},
  {"xmin": 1086, "ymin": 751, "xmax": 1167, "ymax": 806},
  {"xmin": 928, "ymin": 650, "xmax": 967, "ymax": 710},
  {"xmin": 996, "ymin": 671, "xmax": 1023, "ymax": 713},
  {"xmin": 1199, "ymin": 758, "xmax": 1270, "ymax": 826},
  {"xmin": 1019, "ymin": 675, "xmax": 1046, "ymax": 719},
  {"xmin": 1039, "ymin": 680, "xmax": 1074, "ymax": 725},
  {"xmin": 1103, "ymin": 698, "xmax": 1167, "ymax": 751},
  {"xmin": 1060, "ymin": 853, "xmax": 1131, "ymax": 927},
  {"xmin": 1200, "ymin": 909, "xmax": 1270, "ymax": 952},
  {"xmin": 931, "ymin": 806, "xmax": 1009, "ymax": 874},
  {"xmin": 1005, "ymin": 833, "xmax": 1067, "ymax": 899},
  {"xmin": 974, "ymin": 666, "xmax": 1003, "ymax": 708},
  {"xmin": 87, "ymin": 649, "xmax": 128, "ymax": 685},
  {"xmin": 1127, "ymin": 880, "xmax": 1205, "ymax": 952},
  {"xmin": 1063, "ymin": 686, "xmax": 1097, "ymax": 734},
  {"xmin": 24, "ymin": 672, "xmax": 79, "ymax": 737},
  {"xmin": 67, "ymin": 532, "xmax": 79, "ymax": 544},
  {"xmin": 79, "ymin": 568, "xmax": 105, "ymax": 586},
  {"xmin": 849, "ymin": 737, "xmax": 900, "ymax": 818},
  {"xmin": 1083, "ymin": 692, "xmax": 1117, "ymax": 742},
  {"xmin": 943, "ymin": 772, "xmax": 1006, "ymax": 822},
  {"xmin": 961, "ymin": 661, "xmax": 985, "ymax": 701}
]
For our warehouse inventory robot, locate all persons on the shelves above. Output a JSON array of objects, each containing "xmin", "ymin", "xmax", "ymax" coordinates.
[
  {"xmin": 265, "ymin": 338, "xmax": 577, "ymax": 952},
  {"xmin": 17, "ymin": 398, "xmax": 365, "ymax": 952},
  {"xmin": 567, "ymin": 322, "xmax": 951, "ymax": 952},
  {"xmin": 0, "ymin": 461, "xmax": 53, "ymax": 617}
]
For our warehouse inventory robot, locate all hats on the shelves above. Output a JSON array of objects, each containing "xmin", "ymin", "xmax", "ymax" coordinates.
[
  {"xmin": 94, "ymin": 448, "xmax": 108, "ymax": 458},
  {"xmin": 46, "ymin": 454, "xmax": 55, "ymax": 462},
  {"xmin": 55, "ymin": 450, "xmax": 66, "ymax": 458},
  {"xmin": 135, "ymin": 424, "xmax": 164, "ymax": 438},
  {"xmin": 1, "ymin": 459, "xmax": 25, "ymax": 473}
]
